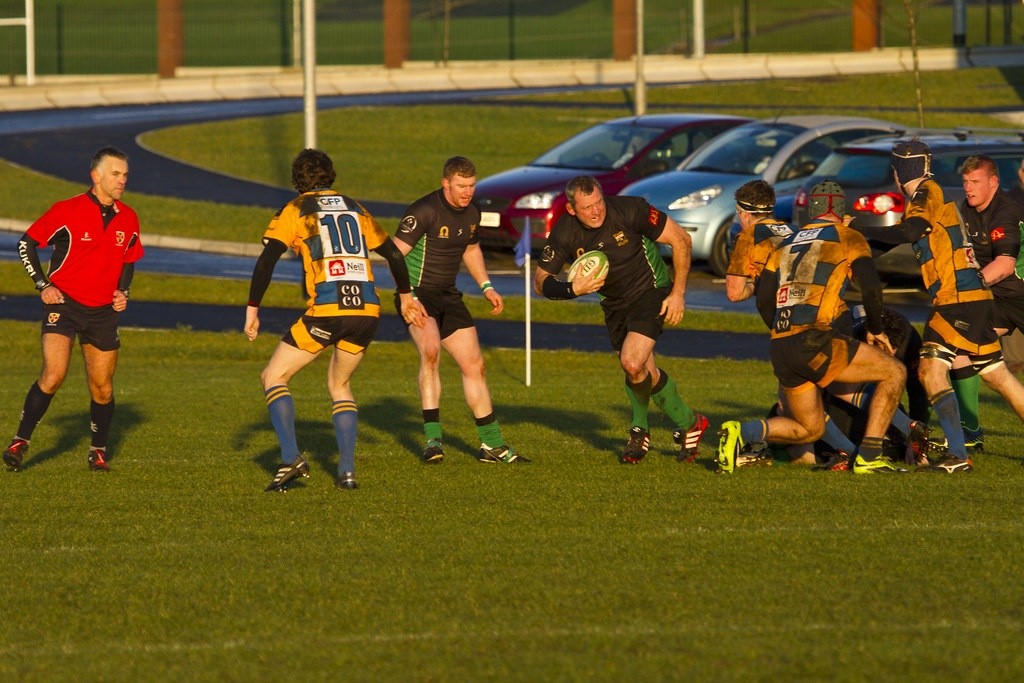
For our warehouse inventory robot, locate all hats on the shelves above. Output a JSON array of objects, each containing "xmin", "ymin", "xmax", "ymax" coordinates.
[
  {"xmin": 808, "ymin": 181, "xmax": 845, "ymax": 220},
  {"xmin": 891, "ymin": 141, "xmax": 932, "ymax": 187}
]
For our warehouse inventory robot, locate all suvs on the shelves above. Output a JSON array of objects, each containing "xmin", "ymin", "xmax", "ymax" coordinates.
[{"xmin": 793, "ymin": 133, "xmax": 1024, "ymax": 231}]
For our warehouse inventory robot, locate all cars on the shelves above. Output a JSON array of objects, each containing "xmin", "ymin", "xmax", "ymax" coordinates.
[
  {"xmin": 618, "ymin": 116, "xmax": 918, "ymax": 279},
  {"xmin": 469, "ymin": 114, "xmax": 751, "ymax": 252}
]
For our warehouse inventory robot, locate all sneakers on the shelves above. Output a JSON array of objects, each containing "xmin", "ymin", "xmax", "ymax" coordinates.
[
  {"xmin": 853, "ymin": 452, "xmax": 908, "ymax": 475},
  {"xmin": 4, "ymin": 434, "xmax": 32, "ymax": 466},
  {"xmin": 927, "ymin": 427, "xmax": 984, "ymax": 452},
  {"xmin": 737, "ymin": 451, "xmax": 773, "ymax": 466},
  {"xmin": 87, "ymin": 445, "xmax": 108, "ymax": 471},
  {"xmin": 264, "ymin": 454, "xmax": 309, "ymax": 492},
  {"xmin": 717, "ymin": 421, "xmax": 746, "ymax": 475},
  {"xmin": 766, "ymin": 401, "xmax": 782, "ymax": 418},
  {"xmin": 673, "ymin": 413, "xmax": 709, "ymax": 463},
  {"xmin": 480, "ymin": 443, "xmax": 530, "ymax": 464},
  {"xmin": 910, "ymin": 421, "xmax": 929, "ymax": 465},
  {"xmin": 621, "ymin": 426, "xmax": 650, "ymax": 463},
  {"xmin": 338, "ymin": 472, "xmax": 357, "ymax": 490},
  {"xmin": 424, "ymin": 439, "xmax": 444, "ymax": 462},
  {"xmin": 914, "ymin": 456, "xmax": 973, "ymax": 474}
]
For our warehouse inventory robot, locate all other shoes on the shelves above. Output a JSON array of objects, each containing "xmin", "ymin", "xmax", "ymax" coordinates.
[{"xmin": 812, "ymin": 449, "xmax": 853, "ymax": 471}]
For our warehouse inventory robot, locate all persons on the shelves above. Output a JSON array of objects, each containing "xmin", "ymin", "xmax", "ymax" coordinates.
[
  {"xmin": 2, "ymin": 148, "xmax": 144, "ymax": 473},
  {"xmin": 244, "ymin": 148, "xmax": 429, "ymax": 492},
  {"xmin": 393, "ymin": 155, "xmax": 532, "ymax": 464},
  {"xmin": 718, "ymin": 141, "xmax": 1024, "ymax": 473},
  {"xmin": 533, "ymin": 174, "xmax": 712, "ymax": 465}
]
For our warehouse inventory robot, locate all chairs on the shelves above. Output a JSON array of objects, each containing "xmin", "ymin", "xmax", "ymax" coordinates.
[{"xmin": 649, "ymin": 134, "xmax": 673, "ymax": 168}]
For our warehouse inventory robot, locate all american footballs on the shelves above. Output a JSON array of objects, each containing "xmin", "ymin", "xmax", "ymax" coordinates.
[{"xmin": 566, "ymin": 249, "xmax": 609, "ymax": 291}]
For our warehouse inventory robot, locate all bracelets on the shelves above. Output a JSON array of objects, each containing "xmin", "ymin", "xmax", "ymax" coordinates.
[
  {"xmin": 480, "ymin": 281, "xmax": 494, "ymax": 294},
  {"xmin": 411, "ymin": 286, "xmax": 418, "ymax": 300}
]
